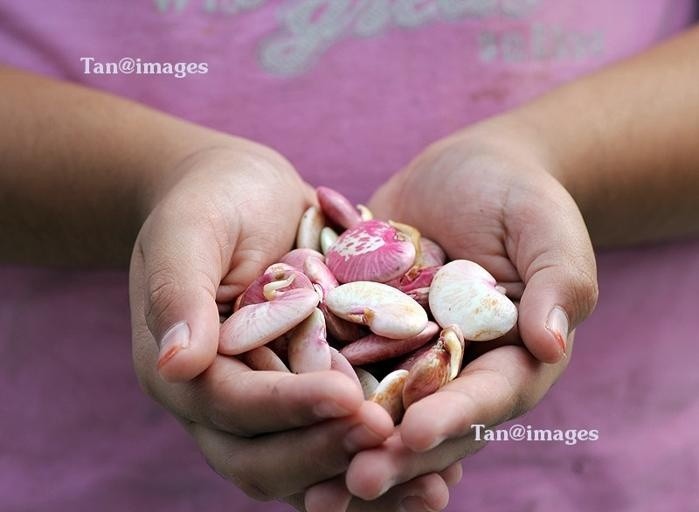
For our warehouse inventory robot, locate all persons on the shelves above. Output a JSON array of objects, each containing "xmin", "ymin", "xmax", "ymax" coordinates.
[{"xmin": 0, "ymin": 0, "xmax": 699, "ymax": 512}]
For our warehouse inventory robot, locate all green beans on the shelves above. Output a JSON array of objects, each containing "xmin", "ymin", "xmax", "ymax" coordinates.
[{"xmin": 218, "ymin": 186, "xmax": 518, "ymax": 423}]
